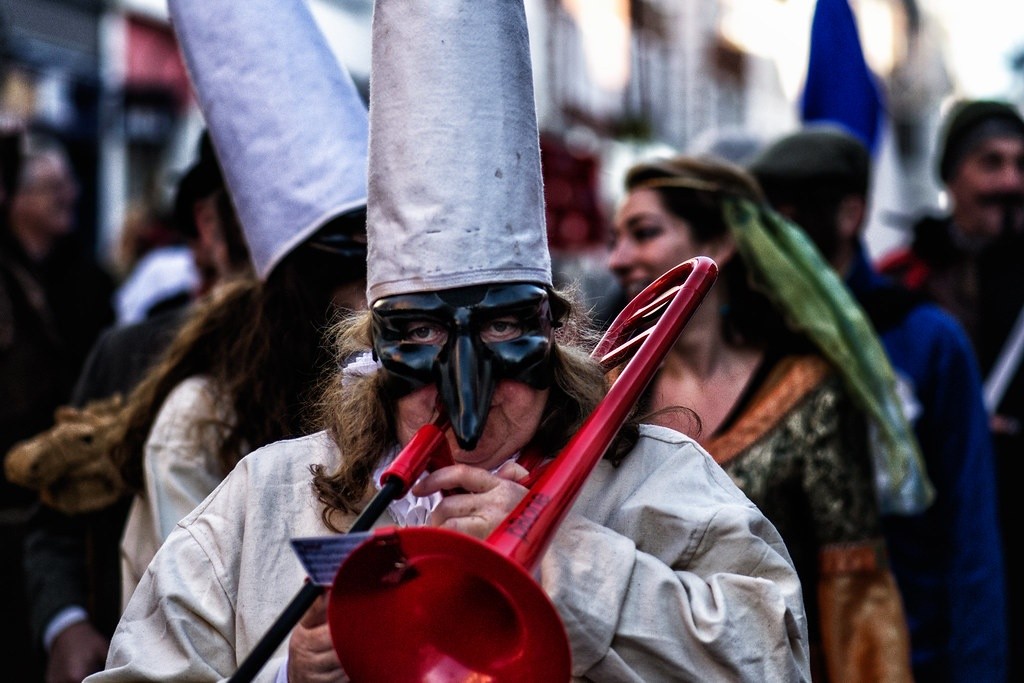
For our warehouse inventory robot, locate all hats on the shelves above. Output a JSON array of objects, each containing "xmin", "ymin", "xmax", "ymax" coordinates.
[
  {"xmin": 938, "ymin": 96, "xmax": 1023, "ymax": 181},
  {"xmin": 165, "ymin": 1, "xmax": 366, "ymax": 280},
  {"xmin": 368, "ymin": 0, "xmax": 554, "ymax": 303}
]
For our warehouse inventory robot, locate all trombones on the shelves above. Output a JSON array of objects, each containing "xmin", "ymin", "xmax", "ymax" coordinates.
[{"xmin": 228, "ymin": 256, "xmax": 719, "ymax": 683}]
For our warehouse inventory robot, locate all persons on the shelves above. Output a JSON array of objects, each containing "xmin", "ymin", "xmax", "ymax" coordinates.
[
  {"xmin": 113, "ymin": 147, "xmax": 208, "ymax": 329},
  {"xmin": 541, "ymin": 124, "xmax": 631, "ymax": 327},
  {"xmin": 195, "ymin": 163, "xmax": 369, "ymax": 486},
  {"xmin": 14, "ymin": 131, "xmax": 256, "ymax": 683},
  {"xmin": 86, "ymin": 238, "xmax": 811, "ymax": 683},
  {"xmin": 876, "ymin": 97, "xmax": 1024, "ymax": 683},
  {"xmin": 607, "ymin": 155, "xmax": 932, "ymax": 683},
  {"xmin": 744, "ymin": 129, "xmax": 1016, "ymax": 681},
  {"xmin": 103, "ymin": 268, "xmax": 261, "ymax": 620},
  {"xmin": 2, "ymin": 112, "xmax": 117, "ymax": 683}
]
